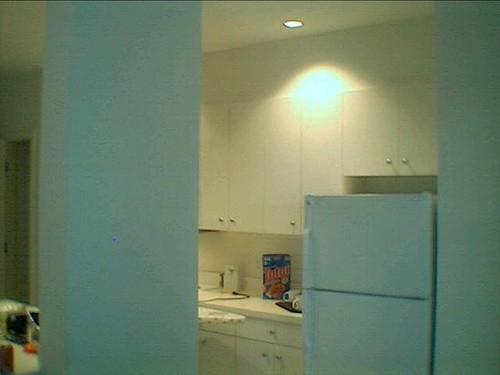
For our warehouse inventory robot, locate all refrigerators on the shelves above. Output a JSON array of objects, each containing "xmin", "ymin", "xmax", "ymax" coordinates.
[{"xmin": 303, "ymin": 192, "xmax": 433, "ymax": 374}]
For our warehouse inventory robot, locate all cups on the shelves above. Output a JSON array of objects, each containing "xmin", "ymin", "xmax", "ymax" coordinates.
[
  {"xmin": 292, "ymin": 295, "xmax": 302, "ymax": 310},
  {"xmin": 223, "ymin": 265, "xmax": 239, "ymax": 293},
  {"xmin": 282, "ymin": 288, "xmax": 300, "ymax": 305}
]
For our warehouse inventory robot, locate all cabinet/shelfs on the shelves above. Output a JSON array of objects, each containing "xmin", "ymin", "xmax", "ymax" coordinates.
[
  {"xmin": 235, "ymin": 318, "xmax": 309, "ymax": 375},
  {"xmin": 339, "ymin": 85, "xmax": 436, "ymax": 178},
  {"xmin": 198, "ymin": 309, "xmax": 237, "ymax": 375},
  {"xmin": 198, "ymin": 99, "xmax": 302, "ymax": 240}
]
